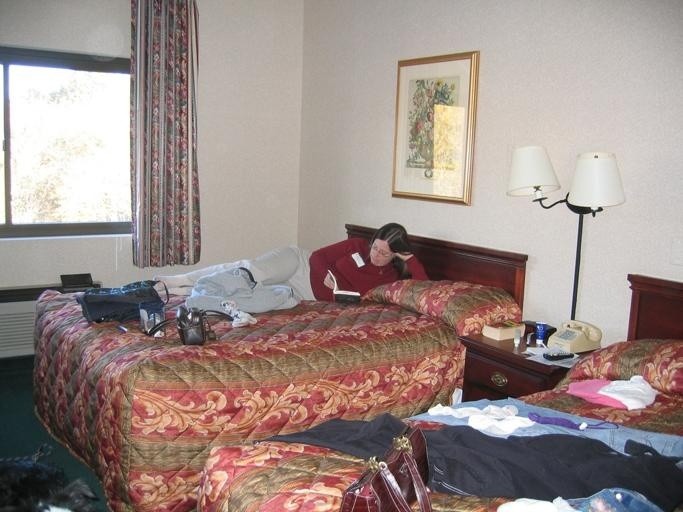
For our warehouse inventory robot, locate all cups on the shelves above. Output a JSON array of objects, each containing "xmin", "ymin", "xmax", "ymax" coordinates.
[{"xmin": 536, "ymin": 322, "xmax": 546, "ymax": 344}]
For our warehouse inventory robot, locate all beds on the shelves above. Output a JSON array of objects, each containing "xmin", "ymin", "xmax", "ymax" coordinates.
[
  {"xmin": 33, "ymin": 226, "xmax": 528, "ymax": 511},
  {"xmin": 193, "ymin": 274, "xmax": 683, "ymax": 512}
]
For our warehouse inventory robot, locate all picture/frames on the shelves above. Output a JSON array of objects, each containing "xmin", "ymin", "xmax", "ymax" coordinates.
[{"xmin": 391, "ymin": 50, "xmax": 480, "ymax": 205}]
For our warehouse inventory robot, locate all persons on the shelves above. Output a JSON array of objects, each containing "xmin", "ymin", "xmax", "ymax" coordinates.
[{"xmin": 153, "ymin": 223, "xmax": 428, "ymax": 313}]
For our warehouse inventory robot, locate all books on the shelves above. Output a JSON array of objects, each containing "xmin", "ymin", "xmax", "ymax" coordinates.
[
  {"xmin": 328, "ymin": 270, "xmax": 361, "ymax": 303},
  {"xmin": 483, "ymin": 320, "xmax": 526, "ymax": 340}
]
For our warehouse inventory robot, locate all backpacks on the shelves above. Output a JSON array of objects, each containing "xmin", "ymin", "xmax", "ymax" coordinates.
[{"xmin": 76, "ymin": 280, "xmax": 169, "ymax": 322}]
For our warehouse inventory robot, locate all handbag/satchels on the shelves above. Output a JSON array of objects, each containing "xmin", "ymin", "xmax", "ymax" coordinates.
[
  {"xmin": 339, "ymin": 425, "xmax": 429, "ymax": 512},
  {"xmin": 176, "ymin": 306, "xmax": 206, "ymax": 345}
]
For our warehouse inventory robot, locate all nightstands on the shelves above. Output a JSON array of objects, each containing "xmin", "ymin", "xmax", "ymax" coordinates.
[{"xmin": 456, "ymin": 334, "xmax": 568, "ymax": 402}]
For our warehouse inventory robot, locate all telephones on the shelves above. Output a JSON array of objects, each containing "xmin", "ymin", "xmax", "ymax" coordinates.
[{"xmin": 547, "ymin": 320, "xmax": 602, "ymax": 354}]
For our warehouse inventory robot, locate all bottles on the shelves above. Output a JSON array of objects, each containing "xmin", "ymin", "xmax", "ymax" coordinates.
[{"xmin": 514, "ymin": 329, "xmax": 520, "ymax": 346}]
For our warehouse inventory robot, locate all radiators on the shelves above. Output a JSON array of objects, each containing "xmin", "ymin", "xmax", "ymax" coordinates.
[{"xmin": 2, "ymin": 284, "xmax": 102, "ymax": 358}]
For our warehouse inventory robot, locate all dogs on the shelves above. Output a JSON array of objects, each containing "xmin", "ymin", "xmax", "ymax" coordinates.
[{"xmin": 0, "ymin": 440, "xmax": 101, "ymax": 512}]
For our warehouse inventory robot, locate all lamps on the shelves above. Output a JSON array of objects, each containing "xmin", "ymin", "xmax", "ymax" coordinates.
[{"xmin": 505, "ymin": 146, "xmax": 630, "ymax": 321}]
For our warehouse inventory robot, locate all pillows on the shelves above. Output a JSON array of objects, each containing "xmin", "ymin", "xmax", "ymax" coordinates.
[
  {"xmin": 363, "ymin": 280, "xmax": 523, "ymax": 335},
  {"xmin": 552, "ymin": 338, "xmax": 683, "ymax": 395}
]
